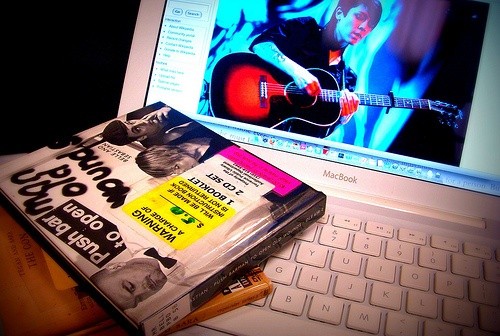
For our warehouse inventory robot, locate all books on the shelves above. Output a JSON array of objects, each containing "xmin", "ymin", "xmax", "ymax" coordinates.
[{"xmin": 0, "ymin": 100, "xmax": 327, "ymax": 336}]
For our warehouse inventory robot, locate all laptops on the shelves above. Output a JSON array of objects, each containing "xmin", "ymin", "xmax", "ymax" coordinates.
[{"xmin": 118, "ymin": 0, "xmax": 500, "ymax": 336}]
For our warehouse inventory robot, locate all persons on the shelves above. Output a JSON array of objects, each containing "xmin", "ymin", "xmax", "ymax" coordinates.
[
  {"xmin": 89, "ymin": 217, "xmax": 195, "ymax": 311},
  {"xmin": 134, "ymin": 119, "xmax": 234, "ymax": 181},
  {"xmin": 96, "ymin": 100, "xmax": 204, "ymax": 146},
  {"xmin": 249, "ymin": 0, "xmax": 382, "ymax": 139}
]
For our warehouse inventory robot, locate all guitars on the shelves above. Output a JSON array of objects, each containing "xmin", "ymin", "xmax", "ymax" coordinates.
[{"xmin": 208, "ymin": 53, "xmax": 464, "ymax": 139}]
